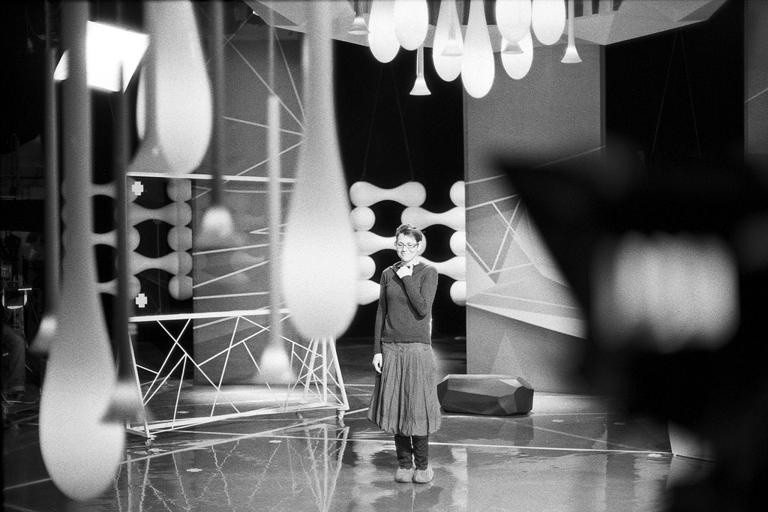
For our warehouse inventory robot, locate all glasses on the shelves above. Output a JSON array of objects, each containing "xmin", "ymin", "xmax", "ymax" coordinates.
[{"xmin": 394, "ymin": 242, "xmax": 419, "ymax": 249}]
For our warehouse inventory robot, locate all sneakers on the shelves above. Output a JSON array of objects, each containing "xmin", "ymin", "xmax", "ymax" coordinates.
[
  {"xmin": 413, "ymin": 466, "xmax": 434, "ymax": 483},
  {"xmin": 396, "ymin": 466, "xmax": 413, "ymax": 483}
]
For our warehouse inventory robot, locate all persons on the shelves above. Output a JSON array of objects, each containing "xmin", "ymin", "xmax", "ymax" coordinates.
[
  {"xmin": 0, "ymin": 323, "xmax": 25, "ymax": 400},
  {"xmin": 365, "ymin": 222, "xmax": 442, "ymax": 485}
]
